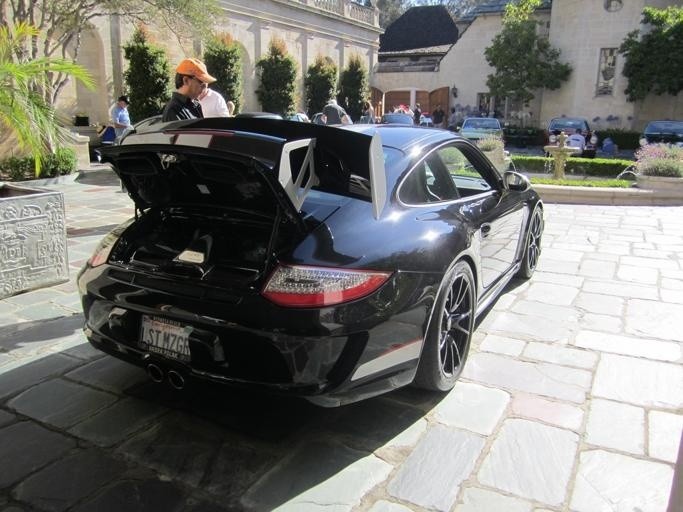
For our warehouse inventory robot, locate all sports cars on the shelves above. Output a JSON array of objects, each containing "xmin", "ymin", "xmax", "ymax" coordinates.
[{"xmin": 75, "ymin": 111, "xmax": 544, "ymax": 408}]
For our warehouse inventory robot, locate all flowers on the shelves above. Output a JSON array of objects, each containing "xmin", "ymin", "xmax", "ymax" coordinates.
[{"xmin": 634, "ymin": 143, "xmax": 683, "ymax": 177}]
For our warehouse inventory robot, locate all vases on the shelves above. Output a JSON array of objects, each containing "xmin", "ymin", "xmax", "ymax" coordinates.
[{"xmin": 635, "ymin": 175, "xmax": 683, "ymax": 190}]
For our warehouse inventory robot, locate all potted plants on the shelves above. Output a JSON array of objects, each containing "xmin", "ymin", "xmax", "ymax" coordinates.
[
  {"xmin": 440, "ymin": 147, "xmax": 466, "ymax": 170},
  {"xmin": 475, "ymin": 135, "xmax": 504, "ymax": 164}
]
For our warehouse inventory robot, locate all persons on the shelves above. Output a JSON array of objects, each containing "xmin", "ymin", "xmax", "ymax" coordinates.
[
  {"xmin": 548, "ymin": 128, "xmax": 584, "ymax": 157},
  {"xmin": 110, "ymin": 95, "xmax": 133, "ymax": 194},
  {"xmin": 190, "ymin": 57, "xmax": 229, "ymax": 120},
  {"xmin": 290, "ymin": 98, "xmax": 481, "ymax": 131},
  {"xmin": 159, "ymin": 58, "xmax": 217, "ymax": 124}
]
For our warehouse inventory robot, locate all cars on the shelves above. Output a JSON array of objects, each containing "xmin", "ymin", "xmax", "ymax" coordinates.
[
  {"xmin": 541, "ymin": 117, "xmax": 597, "ymax": 157},
  {"xmin": 378, "ymin": 113, "xmax": 414, "ymax": 126},
  {"xmin": 113, "ymin": 111, "xmax": 284, "ymax": 147},
  {"xmin": 638, "ymin": 119, "xmax": 683, "ymax": 148},
  {"xmin": 456, "ymin": 117, "xmax": 506, "ymax": 150}
]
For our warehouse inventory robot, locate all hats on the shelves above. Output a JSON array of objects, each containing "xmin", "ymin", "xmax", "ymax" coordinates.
[
  {"xmin": 175, "ymin": 58, "xmax": 217, "ymax": 84},
  {"xmin": 119, "ymin": 96, "xmax": 130, "ymax": 104}
]
